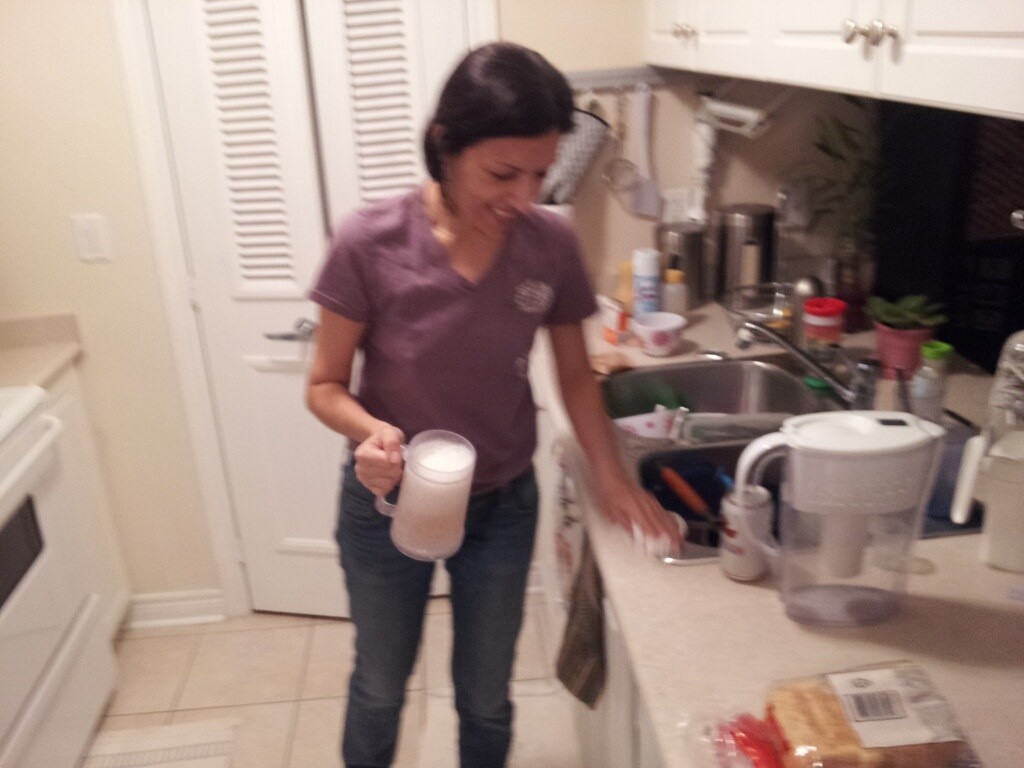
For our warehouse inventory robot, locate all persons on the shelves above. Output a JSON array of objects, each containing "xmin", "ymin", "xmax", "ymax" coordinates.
[{"xmin": 305, "ymin": 44, "xmax": 685, "ymax": 768}]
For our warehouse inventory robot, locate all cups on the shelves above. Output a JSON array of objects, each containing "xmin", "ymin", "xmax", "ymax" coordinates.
[
  {"xmin": 907, "ymin": 341, "xmax": 953, "ymax": 418},
  {"xmin": 721, "ymin": 204, "xmax": 776, "ymax": 310},
  {"xmin": 373, "ymin": 428, "xmax": 477, "ymax": 562},
  {"xmin": 658, "ymin": 220, "xmax": 704, "ymax": 308}
]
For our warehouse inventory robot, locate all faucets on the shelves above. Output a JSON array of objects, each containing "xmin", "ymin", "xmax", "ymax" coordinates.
[{"xmin": 734, "ymin": 321, "xmax": 879, "ymax": 410}]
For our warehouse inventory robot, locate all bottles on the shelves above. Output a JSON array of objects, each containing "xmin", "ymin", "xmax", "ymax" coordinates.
[
  {"xmin": 658, "ymin": 269, "xmax": 688, "ymax": 319},
  {"xmin": 631, "ymin": 247, "xmax": 662, "ymax": 312}
]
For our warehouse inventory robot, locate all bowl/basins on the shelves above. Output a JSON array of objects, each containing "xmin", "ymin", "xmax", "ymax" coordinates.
[
  {"xmin": 630, "ymin": 312, "xmax": 689, "ymax": 357},
  {"xmin": 611, "ymin": 409, "xmax": 676, "ymax": 439}
]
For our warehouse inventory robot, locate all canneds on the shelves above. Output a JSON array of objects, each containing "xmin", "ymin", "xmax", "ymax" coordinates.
[{"xmin": 720, "ymin": 484, "xmax": 774, "ymax": 582}]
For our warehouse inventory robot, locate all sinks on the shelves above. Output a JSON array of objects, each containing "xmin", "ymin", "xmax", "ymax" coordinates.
[
  {"xmin": 584, "ymin": 358, "xmax": 849, "ymax": 442},
  {"xmin": 614, "ymin": 439, "xmax": 983, "ymax": 566}
]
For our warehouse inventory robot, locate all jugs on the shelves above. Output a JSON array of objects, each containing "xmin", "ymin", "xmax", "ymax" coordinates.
[{"xmin": 734, "ymin": 409, "xmax": 948, "ymax": 627}]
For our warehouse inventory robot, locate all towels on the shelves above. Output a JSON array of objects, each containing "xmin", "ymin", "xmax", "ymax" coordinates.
[
  {"xmin": 548, "ymin": 454, "xmax": 584, "ymax": 616},
  {"xmin": 553, "ymin": 537, "xmax": 607, "ymax": 711}
]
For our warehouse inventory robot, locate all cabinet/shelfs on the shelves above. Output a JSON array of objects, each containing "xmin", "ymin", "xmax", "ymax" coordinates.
[{"xmin": 643, "ymin": 0, "xmax": 1023, "ymax": 122}]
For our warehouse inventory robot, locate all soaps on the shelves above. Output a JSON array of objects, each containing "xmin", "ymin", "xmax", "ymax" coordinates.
[{"xmin": 804, "ymin": 360, "xmax": 850, "ymax": 381}]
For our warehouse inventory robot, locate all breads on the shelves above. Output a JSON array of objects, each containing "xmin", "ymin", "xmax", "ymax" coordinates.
[{"xmin": 766, "ymin": 660, "xmax": 974, "ymax": 768}]
